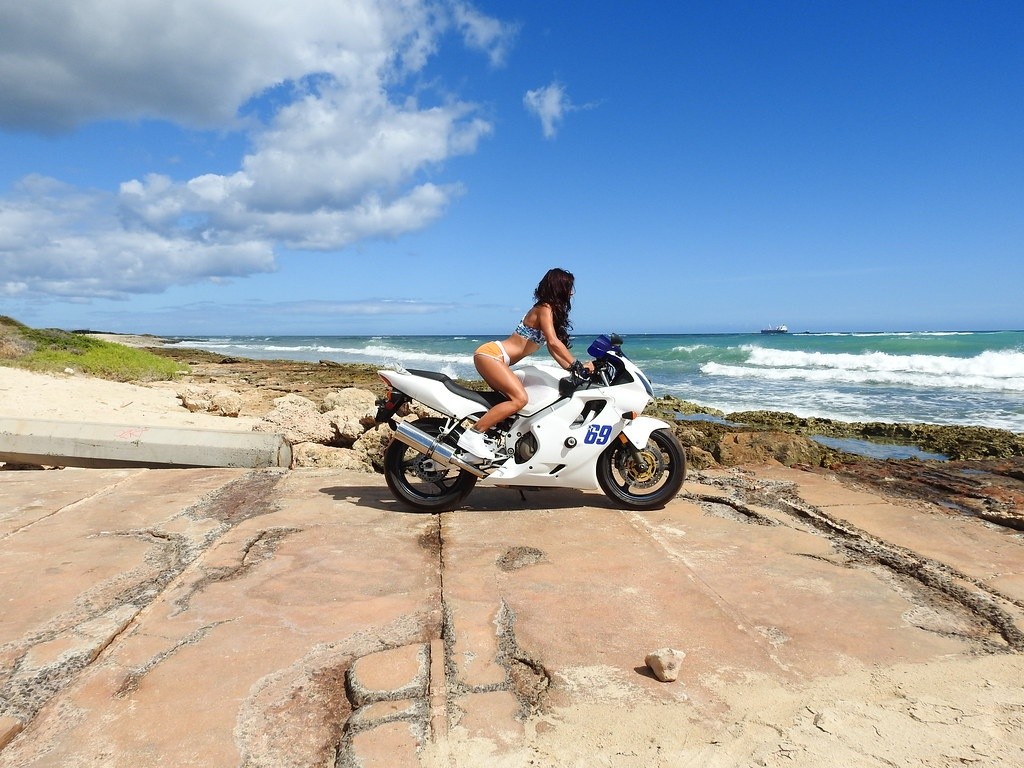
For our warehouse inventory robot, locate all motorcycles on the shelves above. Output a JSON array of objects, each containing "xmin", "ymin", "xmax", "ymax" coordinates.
[{"xmin": 374, "ymin": 331, "xmax": 688, "ymax": 511}]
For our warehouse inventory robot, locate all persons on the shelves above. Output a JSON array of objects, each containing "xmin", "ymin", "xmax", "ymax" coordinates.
[{"xmin": 457, "ymin": 268, "xmax": 595, "ymax": 459}]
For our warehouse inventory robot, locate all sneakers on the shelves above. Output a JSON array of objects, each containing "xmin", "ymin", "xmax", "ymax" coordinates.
[{"xmin": 457, "ymin": 428, "xmax": 495, "ymax": 459}]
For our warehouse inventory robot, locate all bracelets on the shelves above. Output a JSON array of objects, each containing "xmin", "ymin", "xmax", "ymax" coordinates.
[
  {"xmin": 567, "ymin": 365, "xmax": 574, "ymax": 371},
  {"xmin": 572, "ymin": 362, "xmax": 582, "ymax": 370}
]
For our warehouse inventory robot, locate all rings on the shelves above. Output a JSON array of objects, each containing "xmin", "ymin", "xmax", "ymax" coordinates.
[{"xmin": 593, "ymin": 369, "xmax": 594, "ymax": 371}]
